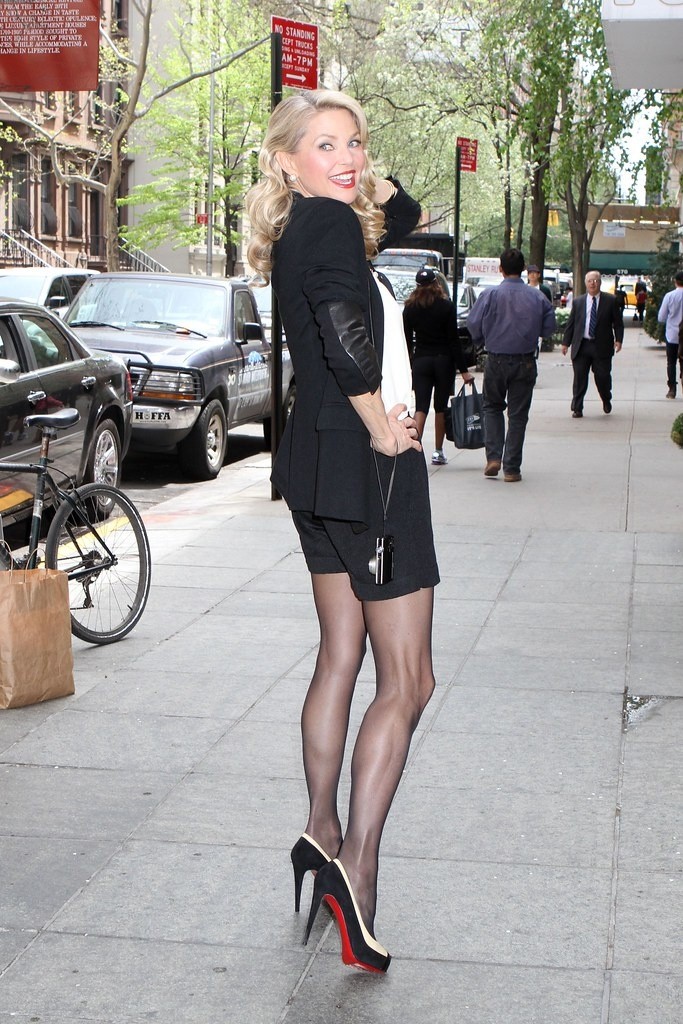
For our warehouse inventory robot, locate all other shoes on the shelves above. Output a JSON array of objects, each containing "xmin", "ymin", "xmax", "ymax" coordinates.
[
  {"xmin": 601, "ymin": 396, "xmax": 612, "ymax": 413},
  {"xmin": 666, "ymin": 388, "xmax": 677, "ymax": 398},
  {"xmin": 485, "ymin": 460, "xmax": 502, "ymax": 476},
  {"xmin": 504, "ymin": 472, "xmax": 522, "ymax": 481},
  {"xmin": 573, "ymin": 409, "xmax": 583, "ymax": 418}
]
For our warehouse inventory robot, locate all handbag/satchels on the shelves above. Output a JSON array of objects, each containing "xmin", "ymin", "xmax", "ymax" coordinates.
[
  {"xmin": 633, "ymin": 314, "xmax": 638, "ymax": 321},
  {"xmin": 0, "ymin": 538, "xmax": 76, "ymax": 710},
  {"xmin": 443, "ymin": 381, "xmax": 485, "ymax": 449}
]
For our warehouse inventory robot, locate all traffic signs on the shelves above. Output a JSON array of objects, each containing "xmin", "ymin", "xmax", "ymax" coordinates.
[
  {"xmin": 456, "ymin": 137, "xmax": 479, "ymax": 173},
  {"xmin": 270, "ymin": 15, "xmax": 318, "ymax": 91}
]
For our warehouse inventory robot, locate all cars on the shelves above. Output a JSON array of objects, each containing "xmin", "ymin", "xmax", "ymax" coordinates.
[
  {"xmin": 610, "ymin": 281, "xmax": 653, "ymax": 305},
  {"xmin": 246, "ymin": 273, "xmax": 287, "ymax": 345},
  {"xmin": 0, "ymin": 267, "xmax": 101, "ymax": 347},
  {"xmin": 0, "ymin": 296, "xmax": 133, "ymax": 528},
  {"xmin": 372, "ymin": 248, "xmax": 574, "ymax": 373}
]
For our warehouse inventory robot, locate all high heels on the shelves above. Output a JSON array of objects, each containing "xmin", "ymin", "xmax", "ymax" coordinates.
[
  {"xmin": 292, "ymin": 835, "xmax": 334, "ymax": 911},
  {"xmin": 301, "ymin": 858, "xmax": 391, "ymax": 975}
]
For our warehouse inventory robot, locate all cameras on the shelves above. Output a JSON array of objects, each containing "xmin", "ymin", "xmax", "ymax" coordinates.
[{"xmin": 368, "ymin": 535, "xmax": 394, "ymax": 584}]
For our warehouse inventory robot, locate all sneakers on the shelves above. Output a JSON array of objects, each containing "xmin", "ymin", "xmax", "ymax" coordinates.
[{"xmin": 432, "ymin": 450, "xmax": 449, "ymax": 464}]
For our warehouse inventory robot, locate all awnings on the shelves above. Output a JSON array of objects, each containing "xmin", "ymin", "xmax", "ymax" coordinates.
[{"xmin": 583, "ymin": 250, "xmax": 674, "ymax": 276}]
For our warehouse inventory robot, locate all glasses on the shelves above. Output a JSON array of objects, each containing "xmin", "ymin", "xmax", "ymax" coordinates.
[{"xmin": 586, "ymin": 279, "xmax": 601, "ymax": 284}]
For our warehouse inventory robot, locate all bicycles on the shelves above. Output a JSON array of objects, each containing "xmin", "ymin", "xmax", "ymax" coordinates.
[{"xmin": 0, "ymin": 407, "xmax": 152, "ymax": 644}]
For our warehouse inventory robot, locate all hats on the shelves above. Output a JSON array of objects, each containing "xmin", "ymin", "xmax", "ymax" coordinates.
[
  {"xmin": 528, "ymin": 265, "xmax": 540, "ymax": 272},
  {"xmin": 415, "ymin": 269, "xmax": 436, "ymax": 285}
]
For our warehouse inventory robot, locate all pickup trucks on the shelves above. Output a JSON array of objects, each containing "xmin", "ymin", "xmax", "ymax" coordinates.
[{"xmin": 61, "ymin": 270, "xmax": 297, "ymax": 484}]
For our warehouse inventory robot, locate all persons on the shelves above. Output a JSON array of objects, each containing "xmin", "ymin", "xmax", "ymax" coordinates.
[
  {"xmin": 613, "ymin": 285, "xmax": 628, "ymax": 328},
  {"xmin": 403, "ymin": 268, "xmax": 476, "ymax": 465},
  {"xmin": 561, "ymin": 270, "xmax": 625, "ymax": 418},
  {"xmin": 562, "ymin": 287, "xmax": 574, "ymax": 310},
  {"xmin": 634, "ymin": 277, "xmax": 648, "ymax": 325},
  {"xmin": 525, "ymin": 265, "xmax": 553, "ymax": 361},
  {"xmin": 468, "ymin": 248, "xmax": 557, "ymax": 482},
  {"xmin": 658, "ymin": 272, "xmax": 683, "ymax": 399},
  {"xmin": 246, "ymin": 91, "xmax": 441, "ymax": 975}
]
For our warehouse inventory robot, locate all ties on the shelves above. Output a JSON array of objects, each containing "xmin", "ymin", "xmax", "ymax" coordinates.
[{"xmin": 589, "ymin": 296, "xmax": 597, "ymax": 337}]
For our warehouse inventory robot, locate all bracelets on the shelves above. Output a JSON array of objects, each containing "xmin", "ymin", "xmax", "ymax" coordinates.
[{"xmin": 378, "ymin": 180, "xmax": 394, "ymax": 205}]
